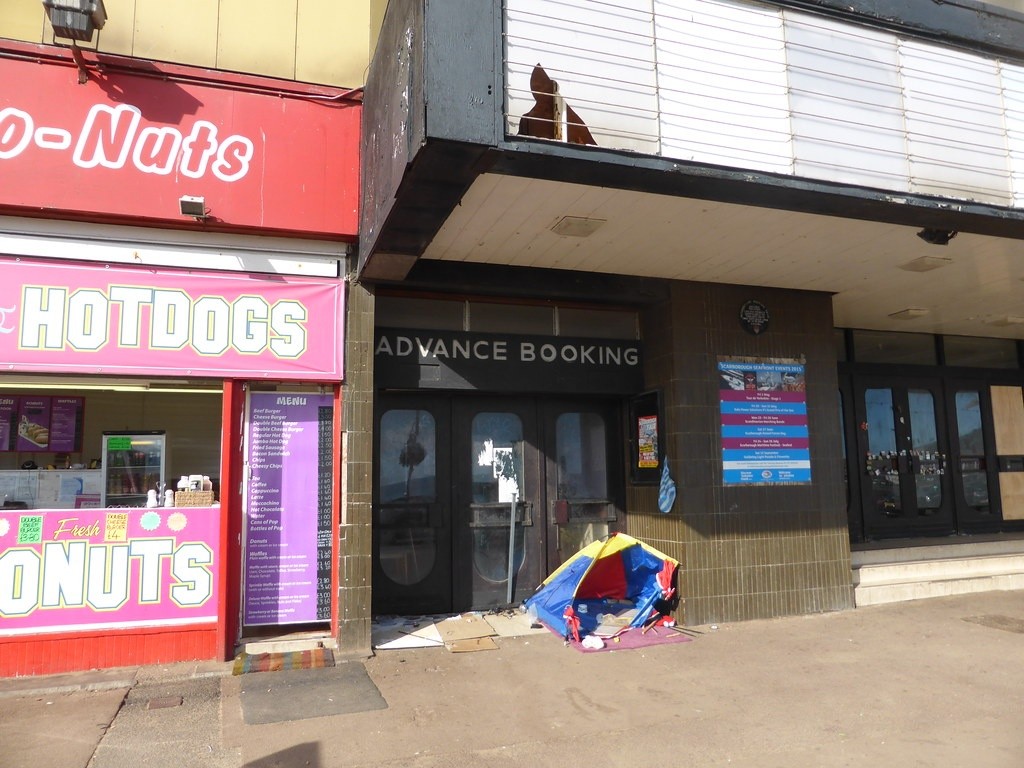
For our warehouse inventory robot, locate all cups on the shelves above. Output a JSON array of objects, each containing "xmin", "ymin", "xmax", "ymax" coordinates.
[{"xmin": 578, "ymin": 604, "xmax": 588, "ymax": 614}]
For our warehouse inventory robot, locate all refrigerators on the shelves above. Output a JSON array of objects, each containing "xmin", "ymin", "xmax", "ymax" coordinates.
[{"xmin": 100, "ymin": 430, "xmax": 167, "ymax": 509}]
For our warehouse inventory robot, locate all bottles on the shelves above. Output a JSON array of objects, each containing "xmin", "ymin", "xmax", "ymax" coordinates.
[
  {"xmin": 107, "ymin": 446, "xmax": 160, "ymax": 467},
  {"xmin": 164, "ymin": 487, "xmax": 175, "ymax": 507},
  {"xmin": 108, "ymin": 469, "xmax": 159, "ymax": 494},
  {"xmin": 177, "ymin": 473, "xmax": 212, "ymax": 492},
  {"xmin": 146, "ymin": 488, "xmax": 158, "ymax": 508}
]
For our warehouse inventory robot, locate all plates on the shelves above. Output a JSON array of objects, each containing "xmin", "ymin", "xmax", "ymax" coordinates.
[{"xmin": 18, "ymin": 422, "xmax": 49, "ymax": 448}]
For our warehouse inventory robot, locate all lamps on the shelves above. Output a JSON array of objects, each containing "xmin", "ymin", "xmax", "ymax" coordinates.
[
  {"xmin": 990, "ymin": 317, "xmax": 1024, "ymax": 327},
  {"xmin": 41, "ymin": 0, "xmax": 108, "ymax": 84},
  {"xmin": 0, "ymin": 382, "xmax": 223, "ymax": 394},
  {"xmin": 917, "ymin": 228, "xmax": 958, "ymax": 246},
  {"xmin": 888, "ymin": 309, "xmax": 930, "ymax": 320},
  {"xmin": 552, "ymin": 217, "xmax": 607, "ymax": 237},
  {"xmin": 899, "ymin": 256, "xmax": 953, "ymax": 272}
]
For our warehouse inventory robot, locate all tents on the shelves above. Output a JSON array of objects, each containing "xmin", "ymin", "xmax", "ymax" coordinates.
[{"xmin": 524, "ymin": 531, "xmax": 682, "ymax": 640}]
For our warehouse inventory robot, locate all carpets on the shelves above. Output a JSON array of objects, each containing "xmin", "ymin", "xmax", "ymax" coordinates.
[
  {"xmin": 242, "ymin": 662, "xmax": 388, "ymax": 725},
  {"xmin": 233, "ymin": 649, "xmax": 337, "ymax": 676}
]
